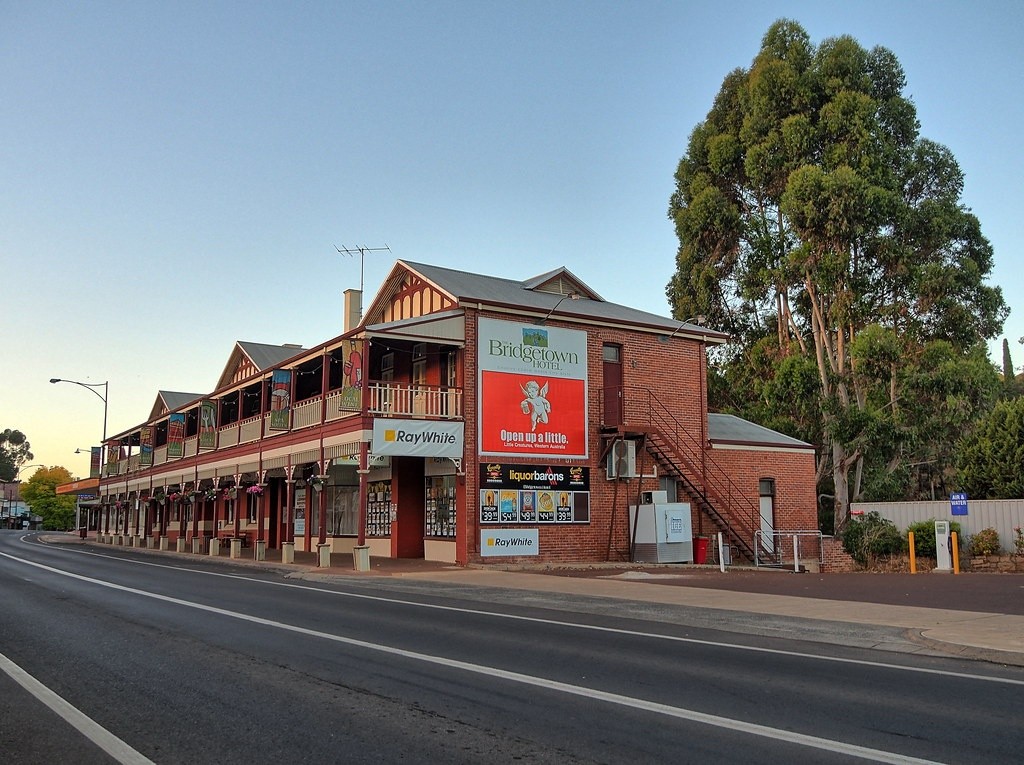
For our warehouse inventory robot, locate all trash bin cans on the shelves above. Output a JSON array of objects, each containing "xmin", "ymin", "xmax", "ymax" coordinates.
[
  {"xmin": 693, "ymin": 537, "xmax": 710, "ymax": 563},
  {"xmin": 80, "ymin": 526, "xmax": 87, "ymax": 537}
]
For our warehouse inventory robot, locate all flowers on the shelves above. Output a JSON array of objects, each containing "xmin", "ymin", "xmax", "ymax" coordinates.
[
  {"xmin": 155, "ymin": 492, "xmax": 166, "ymax": 505},
  {"xmin": 185, "ymin": 491, "xmax": 196, "ymax": 503},
  {"xmin": 306, "ymin": 474, "xmax": 323, "ymax": 491},
  {"xmin": 90, "ymin": 503, "xmax": 104, "ymax": 511},
  {"xmin": 170, "ymin": 493, "xmax": 182, "ymax": 505},
  {"xmin": 202, "ymin": 489, "xmax": 216, "ymax": 500},
  {"xmin": 246, "ymin": 485, "xmax": 263, "ymax": 494},
  {"xmin": 116, "ymin": 499, "xmax": 126, "ymax": 510},
  {"xmin": 140, "ymin": 495, "xmax": 150, "ymax": 507},
  {"xmin": 222, "ymin": 487, "xmax": 235, "ymax": 500}
]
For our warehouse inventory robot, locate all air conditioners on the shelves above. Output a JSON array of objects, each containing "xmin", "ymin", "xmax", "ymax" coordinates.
[{"xmin": 607, "ymin": 440, "xmax": 636, "ymax": 478}]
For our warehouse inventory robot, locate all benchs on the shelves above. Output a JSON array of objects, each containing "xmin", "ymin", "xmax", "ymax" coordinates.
[{"xmin": 221, "ymin": 530, "xmax": 248, "ymax": 548}]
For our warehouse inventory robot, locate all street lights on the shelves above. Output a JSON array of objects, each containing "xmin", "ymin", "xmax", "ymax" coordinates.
[
  {"xmin": 49, "ymin": 379, "xmax": 109, "ymax": 478},
  {"xmin": 14, "ymin": 463, "xmax": 44, "ymax": 529}
]
